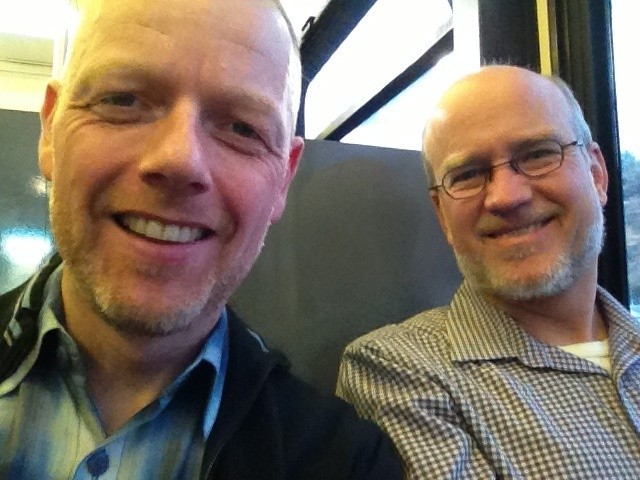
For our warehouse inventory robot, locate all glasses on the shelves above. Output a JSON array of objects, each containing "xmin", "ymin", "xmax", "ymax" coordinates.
[{"xmin": 428, "ymin": 139, "xmax": 584, "ymax": 200}]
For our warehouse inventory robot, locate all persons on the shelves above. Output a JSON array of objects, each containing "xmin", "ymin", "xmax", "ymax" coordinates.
[
  {"xmin": 330, "ymin": 63, "xmax": 640, "ymax": 480},
  {"xmin": 0, "ymin": 0, "xmax": 414, "ymax": 480}
]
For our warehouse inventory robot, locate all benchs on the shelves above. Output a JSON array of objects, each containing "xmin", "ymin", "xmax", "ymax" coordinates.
[{"xmin": 1, "ymin": 110, "xmax": 465, "ymax": 480}]
